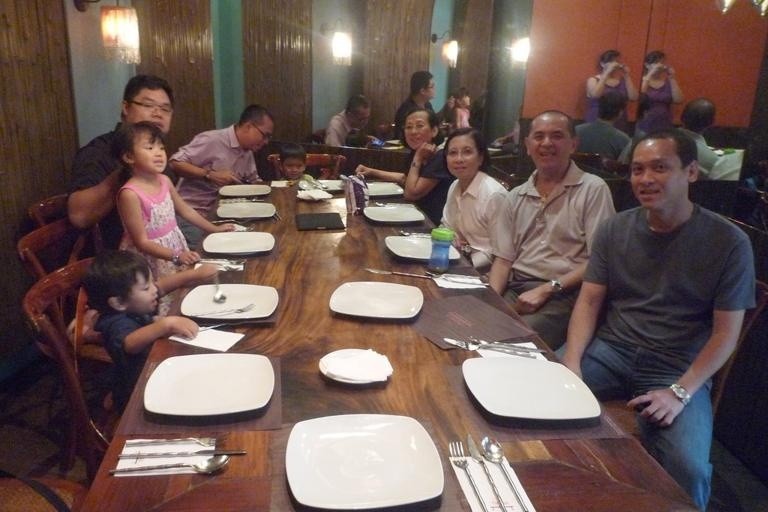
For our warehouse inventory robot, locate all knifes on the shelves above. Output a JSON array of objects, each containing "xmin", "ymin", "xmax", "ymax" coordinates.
[
  {"xmin": 467, "ymin": 432, "xmax": 506, "ymax": 512},
  {"xmin": 119, "ymin": 449, "xmax": 247, "ymax": 460},
  {"xmin": 443, "ymin": 337, "xmax": 548, "ymax": 359},
  {"xmin": 364, "ymin": 267, "xmax": 432, "ymax": 279}
]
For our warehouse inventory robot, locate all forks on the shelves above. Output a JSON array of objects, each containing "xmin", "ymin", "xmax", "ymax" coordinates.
[
  {"xmin": 126, "ymin": 431, "xmax": 232, "ymax": 447},
  {"xmin": 448, "ymin": 441, "xmax": 488, "ymax": 512},
  {"xmin": 186, "ymin": 302, "xmax": 255, "ymax": 319}
]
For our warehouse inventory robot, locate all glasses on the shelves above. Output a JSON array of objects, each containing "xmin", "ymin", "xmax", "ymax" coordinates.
[
  {"xmin": 128, "ymin": 100, "xmax": 173, "ymax": 114},
  {"xmin": 355, "ymin": 114, "xmax": 373, "ymax": 122},
  {"xmin": 250, "ymin": 122, "xmax": 273, "ymax": 139},
  {"xmin": 426, "ymin": 83, "xmax": 436, "ymax": 89}
]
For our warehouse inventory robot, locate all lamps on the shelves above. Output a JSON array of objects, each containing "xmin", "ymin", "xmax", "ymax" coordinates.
[
  {"xmin": 429, "ymin": 28, "xmax": 460, "ymax": 66},
  {"xmin": 75, "ymin": 0, "xmax": 148, "ymax": 64},
  {"xmin": 335, "ymin": 22, "xmax": 366, "ymax": 69}
]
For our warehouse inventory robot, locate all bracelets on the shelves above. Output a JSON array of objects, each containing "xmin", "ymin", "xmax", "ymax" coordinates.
[
  {"xmin": 172, "ymin": 250, "xmax": 182, "ymax": 266},
  {"xmin": 411, "ymin": 161, "xmax": 421, "ymax": 170}
]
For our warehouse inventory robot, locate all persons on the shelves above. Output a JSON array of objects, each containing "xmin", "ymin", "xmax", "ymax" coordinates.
[
  {"xmin": 394, "ymin": 71, "xmax": 456, "ymax": 146},
  {"xmin": 67, "ymin": 74, "xmax": 175, "ymax": 255},
  {"xmin": 487, "ymin": 109, "xmax": 617, "ymax": 351},
  {"xmin": 554, "ymin": 128, "xmax": 757, "ymax": 512},
  {"xmin": 575, "ymin": 91, "xmax": 630, "ymax": 160},
  {"xmin": 356, "ymin": 107, "xmax": 457, "ymax": 228},
  {"xmin": 278, "ymin": 142, "xmax": 314, "ymax": 181},
  {"xmin": 167, "ymin": 104, "xmax": 275, "ymax": 252},
  {"xmin": 437, "ymin": 128, "xmax": 509, "ymax": 281},
  {"xmin": 453, "ymin": 88, "xmax": 471, "ymax": 130},
  {"xmin": 81, "ymin": 249, "xmax": 218, "ymax": 417},
  {"xmin": 585, "ymin": 50, "xmax": 640, "ymax": 136},
  {"xmin": 675, "ymin": 97, "xmax": 720, "ymax": 181},
  {"xmin": 632, "ymin": 93, "xmax": 656, "ymax": 146},
  {"xmin": 112, "ymin": 121, "xmax": 235, "ymax": 316},
  {"xmin": 324, "ymin": 95, "xmax": 380, "ymax": 148},
  {"xmin": 641, "ymin": 51, "xmax": 687, "ymax": 136}
]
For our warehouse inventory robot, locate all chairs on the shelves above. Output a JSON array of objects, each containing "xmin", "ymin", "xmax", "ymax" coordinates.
[
  {"xmin": 569, "ymin": 153, "xmax": 617, "ymax": 176},
  {"xmin": 480, "ymin": 165, "xmax": 513, "ymax": 205},
  {"xmin": 18, "ymin": 215, "xmax": 109, "ymax": 420},
  {"xmin": 28, "ymin": 186, "xmax": 90, "ymax": 243},
  {"xmin": 268, "ymin": 151, "xmax": 349, "ymax": 184},
  {"xmin": 529, "ymin": 176, "xmax": 629, "ymax": 214},
  {"xmin": 603, "ymin": 207, "xmax": 768, "ymax": 450},
  {"xmin": 733, "ymin": 187, "xmax": 768, "ymax": 234},
  {"xmin": 23, "ymin": 253, "xmax": 130, "ymax": 482}
]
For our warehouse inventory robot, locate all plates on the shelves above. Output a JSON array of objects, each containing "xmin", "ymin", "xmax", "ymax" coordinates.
[
  {"xmin": 217, "ymin": 202, "xmax": 276, "ymax": 218},
  {"xmin": 367, "ymin": 182, "xmax": 404, "ymax": 196},
  {"xmin": 299, "ymin": 180, "xmax": 345, "ymax": 191},
  {"xmin": 285, "ymin": 413, "xmax": 444, "ymax": 510},
  {"xmin": 218, "ymin": 185, "xmax": 272, "ymax": 196},
  {"xmin": 462, "ymin": 357, "xmax": 601, "ymax": 421},
  {"xmin": 386, "ymin": 139, "xmax": 400, "ymax": 144},
  {"xmin": 384, "ymin": 235, "xmax": 460, "ymax": 262},
  {"xmin": 318, "ymin": 348, "xmax": 382, "ymax": 383},
  {"xmin": 143, "ymin": 353, "xmax": 275, "ymax": 416},
  {"xmin": 363, "ymin": 207, "xmax": 425, "ymax": 223},
  {"xmin": 329, "ymin": 281, "xmax": 424, "ymax": 319},
  {"xmin": 180, "ymin": 283, "xmax": 279, "ymax": 319},
  {"xmin": 202, "ymin": 231, "xmax": 275, "ymax": 255}
]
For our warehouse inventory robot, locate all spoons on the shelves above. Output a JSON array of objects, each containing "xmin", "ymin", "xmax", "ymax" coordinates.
[
  {"xmin": 482, "ymin": 436, "xmax": 529, "ymax": 511},
  {"xmin": 375, "ymin": 201, "xmax": 394, "ymax": 207},
  {"xmin": 209, "ymin": 270, "xmax": 226, "ymax": 304},
  {"xmin": 399, "ymin": 230, "xmax": 427, "ymax": 236},
  {"xmin": 107, "ymin": 454, "xmax": 231, "ymax": 474}
]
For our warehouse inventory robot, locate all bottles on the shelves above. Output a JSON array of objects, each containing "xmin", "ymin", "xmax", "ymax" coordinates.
[{"xmin": 428, "ymin": 227, "xmax": 454, "ymax": 273}]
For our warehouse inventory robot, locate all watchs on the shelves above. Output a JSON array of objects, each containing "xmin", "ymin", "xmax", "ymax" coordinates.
[
  {"xmin": 202, "ymin": 170, "xmax": 210, "ymax": 183},
  {"xmin": 668, "ymin": 383, "xmax": 691, "ymax": 405},
  {"xmin": 548, "ymin": 276, "xmax": 564, "ymax": 296}
]
